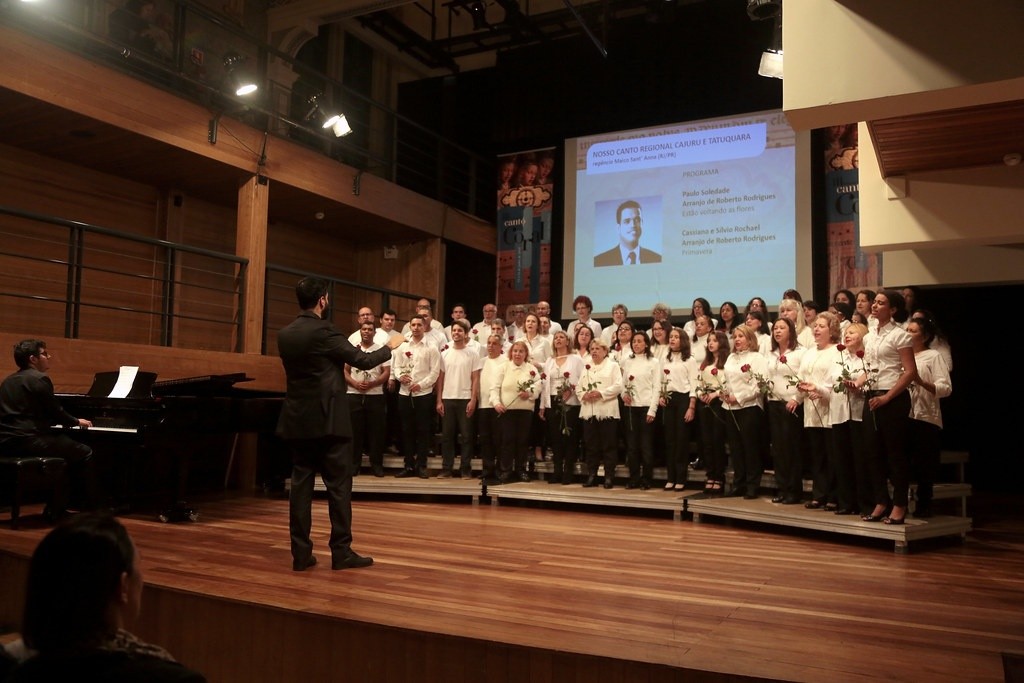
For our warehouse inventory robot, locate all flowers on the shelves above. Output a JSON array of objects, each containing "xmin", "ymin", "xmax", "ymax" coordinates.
[
  {"xmin": 497, "ymin": 371, "xmax": 546, "ymax": 418},
  {"xmin": 833, "ymin": 344, "xmax": 857, "ymax": 422},
  {"xmin": 582, "ymin": 364, "xmax": 601, "ymax": 423},
  {"xmin": 441, "ymin": 344, "xmax": 448, "ymax": 352},
  {"xmin": 659, "ymin": 369, "xmax": 672, "ymax": 424},
  {"xmin": 694, "ymin": 364, "xmax": 726, "ymax": 424},
  {"xmin": 554, "ymin": 372, "xmax": 575, "ymax": 436},
  {"xmin": 400, "ymin": 352, "xmax": 414, "ymax": 409},
  {"xmin": 856, "ymin": 350, "xmax": 879, "ymax": 431},
  {"xmin": 509, "ymin": 336, "xmax": 514, "ymax": 343},
  {"xmin": 614, "ymin": 346, "xmax": 621, "ymax": 366},
  {"xmin": 711, "ymin": 368, "xmax": 740, "ymax": 431},
  {"xmin": 356, "ymin": 344, "xmax": 371, "ymax": 405},
  {"xmin": 472, "ymin": 328, "xmax": 478, "ymax": 342},
  {"xmin": 779, "ymin": 355, "xmax": 823, "ymax": 428},
  {"xmin": 741, "ymin": 363, "xmax": 798, "ymax": 418},
  {"xmin": 624, "ymin": 375, "xmax": 636, "ymax": 430}
]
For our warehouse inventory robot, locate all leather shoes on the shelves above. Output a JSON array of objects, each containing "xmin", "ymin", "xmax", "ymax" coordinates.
[
  {"xmin": 333, "ymin": 556, "xmax": 373, "ymax": 570},
  {"xmin": 293, "ymin": 557, "xmax": 316, "ymax": 570}
]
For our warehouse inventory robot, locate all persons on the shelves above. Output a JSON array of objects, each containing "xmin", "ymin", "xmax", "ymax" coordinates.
[
  {"xmin": 594, "ymin": 200, "xmax": 662, "ymax": 267},
  {"xmin": 823, "ymin": 123, "xmax": 859, "ymax": 170},
  {"xmin": 108, "ymin": 0, "xmax": 175, "ymax": 69},
  {"xmin": 0, "ymin": 339, "xmax": 117, "ymax": 518},
  {"xmin": 0, "ymin": 510, "xmax": 174, "ymax": 661},
  {"xmin": 499, "ymin": 149, "xmax": 555, "ymax": 191},
  {"xmin": 277, "ymin": 275, "xmax": 410, "ymax": 569}
]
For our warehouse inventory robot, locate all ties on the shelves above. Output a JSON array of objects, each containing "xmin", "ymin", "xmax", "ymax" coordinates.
[{"xmin": 629, "ymin": 252, "xmax": 637, "ymax": 265}]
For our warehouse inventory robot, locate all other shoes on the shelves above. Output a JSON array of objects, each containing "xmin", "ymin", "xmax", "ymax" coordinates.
[
  {"xmin": 351, "ymin": 442, "xmax": 930, "ymax": 524},
  {"xmin": 45, "ymin": 504, "xmax": 81, "ymax": 520}
]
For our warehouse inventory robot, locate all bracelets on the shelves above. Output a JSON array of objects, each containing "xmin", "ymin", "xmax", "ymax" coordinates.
[
  {"xmin": 387, "ymin": 342, "xmax": 392, "ymax": 351},
  {"xmin": 689, "ymin": 407, "xmax": 695, "ymax": 409}
]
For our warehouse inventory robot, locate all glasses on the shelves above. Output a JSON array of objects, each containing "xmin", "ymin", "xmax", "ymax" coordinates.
[
  {"xmin": 483, "ymin": 310, "xmax": 495, "ymax": 312},
  {"xmin": 614, "ymin": 311, "xmax": 625, "ymax": 315},
  {"xmin": 516, "ymin": 311, "xmax": 524, "ymax": 314},
  {"xmin": 652, "ymin": 310, "xmax": 664, "ymax": 314},
  {"xmin": 417, "ymin": 305, "xmax": 427, "ymax": 308},
  {"xmin": 619, "ymin": 327, "xmax": 631, "ymax": 331},
  {"xmin": 832, "ymin": 310, "xmax": 843, "ymax": 314},
  {"xmin": 694, "ymin": 305, "xmax": 702, "ymax": 309},
  {"xmin": 750, "ymin": 304, "xmax": 761, "ymax": 307},
  {"xmin": 34, "ymin": 351, "xmax": 48, "ymax": 356},
  {"xmin": 576, "ymin": 307, "xmax": 587, "ymax": 310}
]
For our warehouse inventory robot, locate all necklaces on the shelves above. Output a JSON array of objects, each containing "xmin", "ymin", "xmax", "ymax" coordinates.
[
  {"xmin": 526, "ymin": 333, "xmax": 536, "ymax": 341},
  {"xmin": 452, "ymin": 345, "xmax": 467, "ymax": 350},
  {"xmin": 515, "ymin": 363, "xmax": 522, "ymax": 367}
]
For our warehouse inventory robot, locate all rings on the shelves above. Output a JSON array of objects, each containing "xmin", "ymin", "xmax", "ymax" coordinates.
[
  {"xmin": 594, "ymin": 395, "xmax": 595, "ymax": 397},
  {"xmin": 341, "ymin": 284, "xmax": 952, "ymax": 524},
  {"xmin": 652, "ymin": 419, "xmax": 654, "ymax": 421}
]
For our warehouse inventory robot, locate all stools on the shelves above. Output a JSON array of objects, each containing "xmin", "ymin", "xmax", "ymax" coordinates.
[{"xmin": 0, "ymin": 456, "xmax": 67, "ymax": 530}]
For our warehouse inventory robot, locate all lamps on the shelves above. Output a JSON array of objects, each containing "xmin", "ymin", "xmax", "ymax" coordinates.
[
  {"xmin": 223, "ymin": 51, "xmax": 259, "ymax": 97},
  {"xmin": 303, "ymin": 92, "xmax": 358, "ymax": 138},
  {"xmin": 758, "ymin": 7, "xmax": 783, "ymax": 78}
]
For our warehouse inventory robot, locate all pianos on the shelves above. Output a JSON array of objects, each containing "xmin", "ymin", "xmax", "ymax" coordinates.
[{"xmin": 51, "ymin": 367, "xmax": 290, "ymax": 522}]
